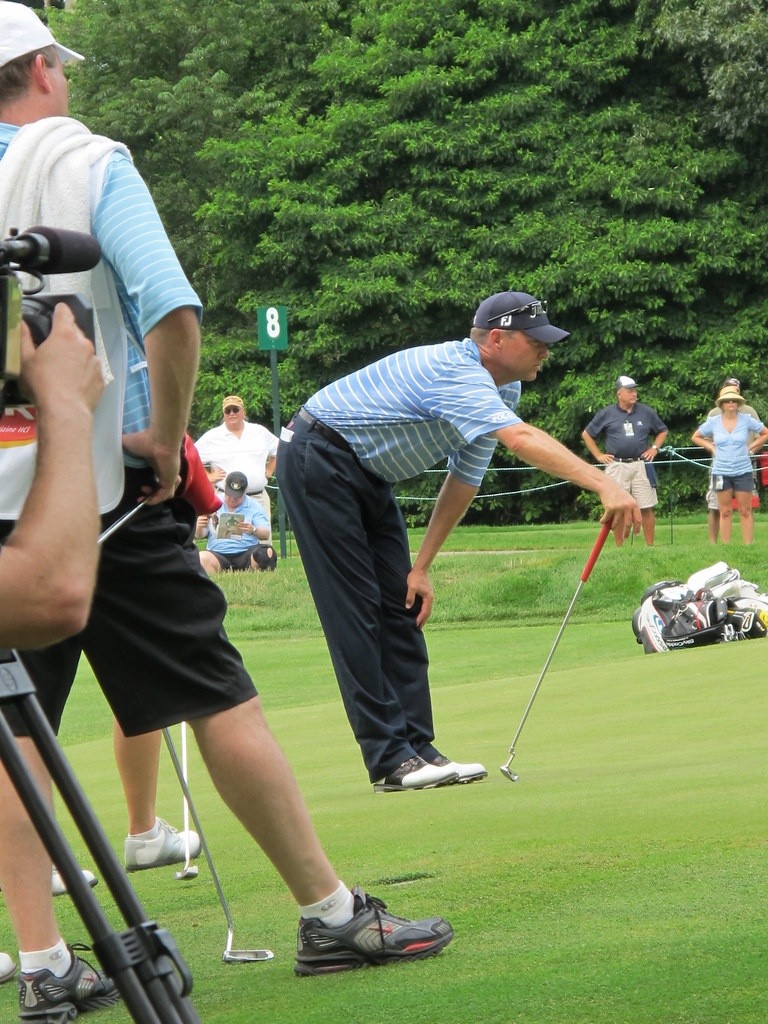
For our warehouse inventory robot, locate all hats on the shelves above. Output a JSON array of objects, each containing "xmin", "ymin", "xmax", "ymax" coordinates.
[
  {"xmin": 222, "ymin": 396, "xmax": 244, "ymax": 409},
  {"xmin": 0, "ymin": 1, "xmax": 85, "ymax": 68},
  {"xmin": 224, "ymin": 472, "xmax": 248, "ymax": 498},
  {"xmin": 715, "ymin": 386, "xmax": 746, "ymax": 408},
  {"xmin": 615, "ymin": 375, "xmax": 638, "ymax": 392},
  {"xmin": 473, "ymin": 290, "xmax": 571, "ymax": 343}
]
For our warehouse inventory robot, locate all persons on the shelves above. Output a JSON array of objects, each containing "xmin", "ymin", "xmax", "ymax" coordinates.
[
  {"xmin": 0, "ymin": 0, "xmax": 458, "ymax": 1024},
  {"xmin": 582, "ymin": 376, "xmax": 668, "ymax": 546},
  {"xmin": 691, "ymin": 376, "xmax": 768, "ymax": 543},
  {"xmin": 274, "ymin": 291, "xmax": 643, "ymax": 792}
]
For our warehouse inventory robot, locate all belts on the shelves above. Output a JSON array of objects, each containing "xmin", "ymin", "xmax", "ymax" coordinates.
[
  {"xmin": 614, "ymin": 456, "xmax": 644, "ymax": 463},
  {"xmin": 298, "ymin": 407, "xmax": 351, "ymax": 452},
  {"xmin": 214, "ymin": 485, "xmax": 263, "ymax": 497}
]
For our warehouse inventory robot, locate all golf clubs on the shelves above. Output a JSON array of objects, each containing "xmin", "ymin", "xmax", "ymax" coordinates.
[
  {"xmin": 162, "ymin": 728, "xmax": 275, "ymax": 963},
  {"xmin": 498, "ymin": 519, "xmax": 613, "ymax": 783},
  {"xmin": 175, "ymin": 721, "xmax": 200, "ymax": 881},
  {"xmin": 99, "ymin": 493, "xmax": 153, "ymax": 545}
]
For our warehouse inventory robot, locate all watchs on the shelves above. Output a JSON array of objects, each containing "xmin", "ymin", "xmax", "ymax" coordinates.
[{"xmin": 652, "ymin": 445, "xmax": 659, "ymax": 451}]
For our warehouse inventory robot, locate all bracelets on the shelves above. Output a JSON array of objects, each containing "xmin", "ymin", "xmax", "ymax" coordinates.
[{"xmin": 750, "ymin": 451, "xmax": 753, "ymax": 455}]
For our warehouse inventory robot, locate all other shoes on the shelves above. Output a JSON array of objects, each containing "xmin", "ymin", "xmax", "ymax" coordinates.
[{"xmin": 0, "ymin": 952, "xmax": 17, "ymax": 983}]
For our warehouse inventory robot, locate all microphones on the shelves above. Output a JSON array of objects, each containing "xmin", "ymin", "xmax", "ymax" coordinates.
[{"xmin": 0, "ymin": 226, "xmax": 102, "ymax": 274}]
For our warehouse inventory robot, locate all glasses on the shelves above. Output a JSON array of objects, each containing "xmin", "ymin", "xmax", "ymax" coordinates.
[
  {"xmin": 724, "ymin": 399, "xmax": 737, "ymax": 403},
  {"xmin": 487, "ymin": 299, "xmax": 550, "ymax": 323},
  {"xmin": 224, "ymin": 407, "xmax": 242, "ymax": 414}
]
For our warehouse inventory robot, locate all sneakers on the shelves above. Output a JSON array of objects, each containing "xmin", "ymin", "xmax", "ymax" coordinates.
[
  {"xmin": 18, "ymin": 943, "xmax": 123, "ymax": 1024},
  {"xmin": 373, "ymin": 755, "xmax": 459, "ymax": 793},
  {"xmin": 52, "ymin": 863, "xmax": 98, "ymax": 896},
  {"xmin": 428, "ymin": 754, "xmax": 488, "ymax": 784},
  {"xmin": 294, "ymin": 885, "xmax": 454, "ymax": 978},
  {"xmin": 124, "ymin": 817, "xmax": 202, "ymax": 873}
]
